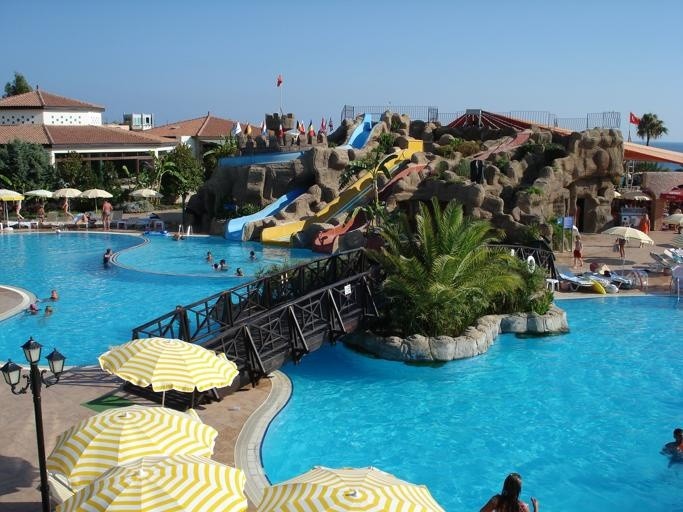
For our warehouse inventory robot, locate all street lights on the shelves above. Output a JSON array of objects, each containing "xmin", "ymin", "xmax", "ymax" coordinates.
[
  {"xmin": 181, "ymin": 192, "xmax": 189, "ymax": 224},
  {"xmin": 0, "ymin": 333, "xmax": 67, "ymax": 512}
]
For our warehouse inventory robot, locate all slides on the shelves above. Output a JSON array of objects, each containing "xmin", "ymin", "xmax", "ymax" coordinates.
[{"xmin": 224, "ymin": 119, "xmax": 399, "ymax": 254}]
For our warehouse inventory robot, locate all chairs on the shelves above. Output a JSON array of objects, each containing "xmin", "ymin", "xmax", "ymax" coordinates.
[
  {"xmin": 110, "ymin": 211, "xmax": 163, "ymax": 230},
  {"xmin": 558, "ymin": 249, "xmax": 683, "ymax": 293}
]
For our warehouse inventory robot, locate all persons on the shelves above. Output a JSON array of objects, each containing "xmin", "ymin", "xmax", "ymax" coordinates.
[
  {"xmin": 665, "ymin": 428, "xmax": 683, "ymax": 455},
  {"xmin": 622, "ymin": 217, "xmax": 628, "ymax": 227},
  {"xmin": 573, "ymin": 235, "xmax": 583, "ymax": 267},
  {"xmin": 674, "ymin": 209, "xmax": 682, "ymax": 231},
  {"xmin": 1, "ymin": 199, "xmax": 257, "ymax": 314},
  {"xmin": 480, "ymin": 472, "xmax": 538, "ymax": 512},
  {"xmin": 639, "ymin": 213, "xmax": 650, "ymax": 248},
  {"xmin": 615, "ymin": 237, "xmax": 626, "ymax": 259}
]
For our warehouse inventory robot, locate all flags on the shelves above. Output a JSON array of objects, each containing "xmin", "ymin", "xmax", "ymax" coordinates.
[
  {"xmin": 630, "ymin": 113, "xmax": 641, "ymax": 124},
  {"xmin": 231, "ymin": 116, "xmax": 326, "ymax": 144},
  {"xmin": 277, "ymin": 74, "xmax": 283, "ymax": 87}
]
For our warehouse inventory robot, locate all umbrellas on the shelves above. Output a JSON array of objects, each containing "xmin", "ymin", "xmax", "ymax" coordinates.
[
  {"xmin": 0, "ymin": 188, "xmax": 164, "ymax": 217},
  {"xmin": 602, "ymin": 226, "xmax": 654, "ymax": 246}
]
